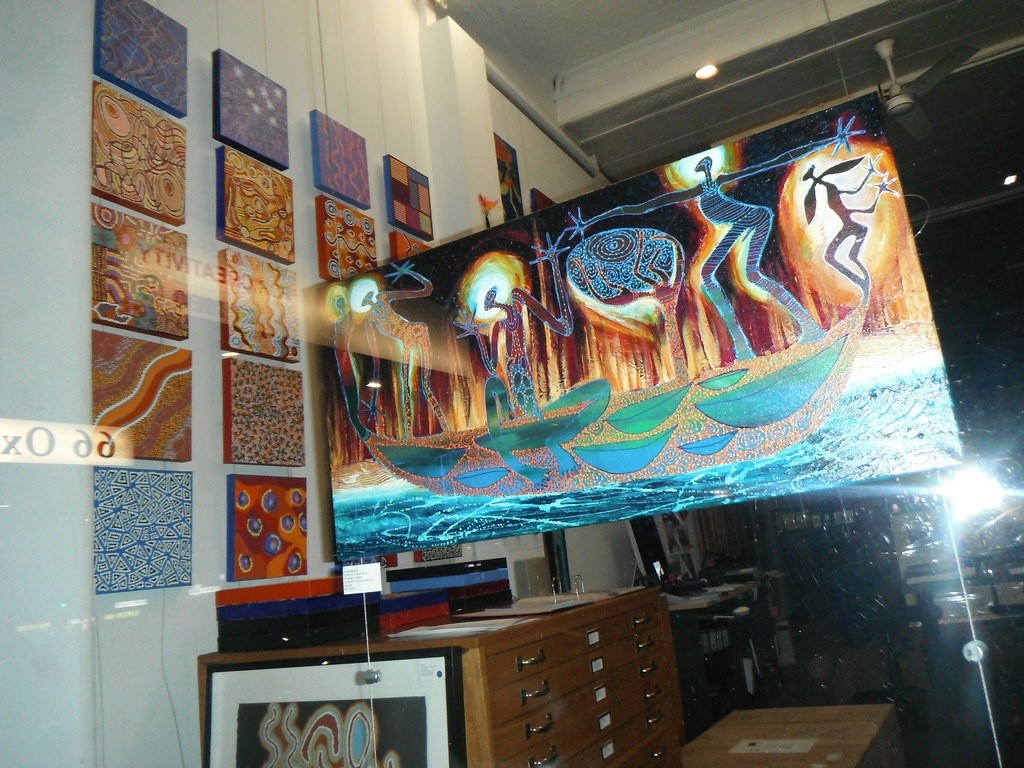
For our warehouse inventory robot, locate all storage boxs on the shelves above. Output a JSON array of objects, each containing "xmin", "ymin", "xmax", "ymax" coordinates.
[{"xmin": 657, "ymin": 701, "xmax": 906, "ymax": 768}]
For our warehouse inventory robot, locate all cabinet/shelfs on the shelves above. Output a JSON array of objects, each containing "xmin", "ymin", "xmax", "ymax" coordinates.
[{"xmin": 198, "ymin": 585, "xmax": 684, "ymax": 768}]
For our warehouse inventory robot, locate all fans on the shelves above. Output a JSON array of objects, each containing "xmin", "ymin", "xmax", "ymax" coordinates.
[{"xmin": 874, "ymin": 35, "xmax": 980, "ymax": 152}]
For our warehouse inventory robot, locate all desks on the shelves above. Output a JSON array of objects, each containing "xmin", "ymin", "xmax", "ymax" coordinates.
[
  {"xmin": 900, "ymin": 548, "xmax": 1024, "ymax": 691},
  {"xmin": 660, "ymin": 570, "xmax": 783, "ymax": 708}
]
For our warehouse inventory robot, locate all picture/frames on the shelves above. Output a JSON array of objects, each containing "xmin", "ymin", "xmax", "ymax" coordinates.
[{"xmin": 202, "ymin": 645, "xmax": 467, "ymax": 768}]
